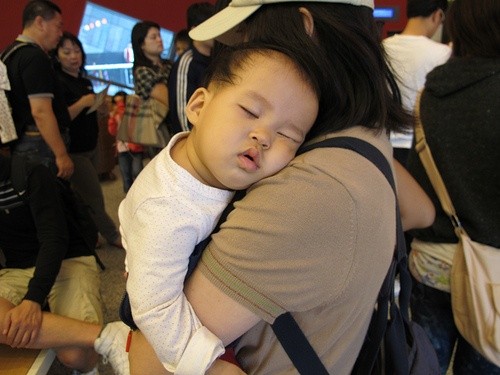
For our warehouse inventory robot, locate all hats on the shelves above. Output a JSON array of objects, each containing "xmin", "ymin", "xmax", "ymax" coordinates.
[{"xmin": 188, "ymin": 0, "xmax": 374, "ymax": 42}]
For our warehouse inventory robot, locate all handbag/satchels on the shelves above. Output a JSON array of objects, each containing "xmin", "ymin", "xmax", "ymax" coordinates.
[
  {"xmin": 408, "ymin": 237, "xmax": 457, "ymax": 293},
  {"xmin": 451, "ymin": 235, "xmax": 500, "ymax": 366},
  {"xmin": 117, "ymin": 67, "xmax": 170, "ymax": 148}
]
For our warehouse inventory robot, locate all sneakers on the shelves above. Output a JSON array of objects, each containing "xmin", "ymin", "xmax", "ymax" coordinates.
[{"xmin": 95, "ymin": 320, "xmax": 132, "ymax": 375}]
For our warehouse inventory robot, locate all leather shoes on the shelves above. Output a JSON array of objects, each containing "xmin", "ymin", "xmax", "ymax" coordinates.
[{"xmin": 109, "ymin": 241, "xmax": 122, "ymax": 248}]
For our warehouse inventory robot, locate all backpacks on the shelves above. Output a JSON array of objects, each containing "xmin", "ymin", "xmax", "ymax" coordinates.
[
  {"xmin": 0, "ymin": 43, "xmax": 29, "ymax": 144},
  {"xmin": 23, "ymin": 161, "xmax": 98, "ymax": 256},
  {"xmin": 273, "ymin": 136, "xmax": 440, "ymax": 375}
]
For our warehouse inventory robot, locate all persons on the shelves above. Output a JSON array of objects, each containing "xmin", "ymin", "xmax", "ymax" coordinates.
[
  {"xmin": 166, "ymin": 2, "xmax": 215, "ymax": 131},
  {"xmin": 377, "ymin": 0, "xmax": 457, "ymax": 280},
  {"xmin": 0, "ymin": 0, "xmax": 123, "ymax": 249},
  {"xmin": 0, "ymin": 0, "xmax": 74, "ymax": 180},
  {"xmin": 130, "ymin": 19, "xmax": 176, "ymax": 154},
  {"xmin": 107, "ymin": 90, "xmax": 148, "ymax": 193},
  {"xmin": 0, "ymin": 149, "xmax": 132, "ymax": 375},
  {"xmin": 128, "ymin": 0, "xmax": 436, "ymax": 375},
  {"xmin": 403, "ymin": 1, "xmax": 500, "ymax": 375},
  {"xmin": 117, "ymin": 39, "xmax": 321, "ymax": 375}
]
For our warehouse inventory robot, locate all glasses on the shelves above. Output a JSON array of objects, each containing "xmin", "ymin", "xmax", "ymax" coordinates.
[{"xmin": 440, "ymin": 10, "xmax": 445, "ymax": 23}]
